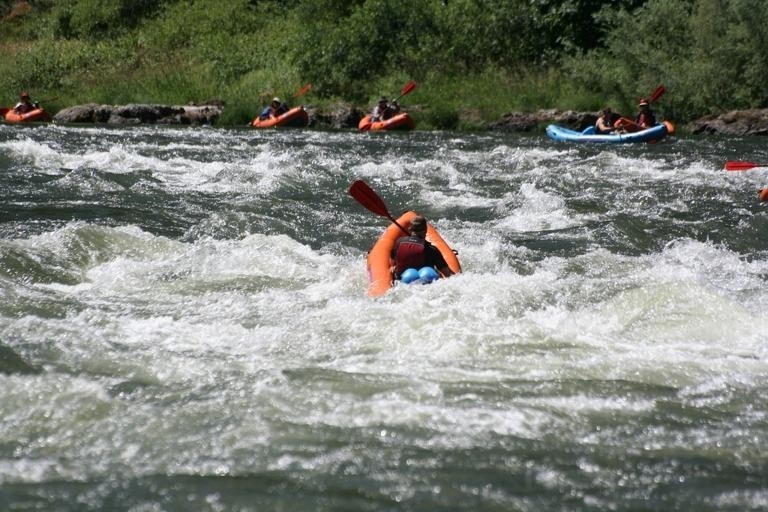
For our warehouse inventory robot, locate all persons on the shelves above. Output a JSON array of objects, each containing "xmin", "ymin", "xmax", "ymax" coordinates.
[
  {"xmin": 257, "ymin": 96, "xmax": 289, "ymax": 122},
  {"xmin": 386, "ymin": 217, "xmax": 448, "ymax": 285},
  {"xmin": 13, "ymin": 91, "xmax": 39, "ymax": 115},
  {"xmin": 594, "ymin": 99, "xmax": 656, "ymax": 135},
  {"xmin": 371, "ymin": 95, "xmax": 401, "ymax": 124}
]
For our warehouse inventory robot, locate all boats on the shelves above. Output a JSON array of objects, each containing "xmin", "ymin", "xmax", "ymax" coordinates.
[
  {"xmin": 249, "ymin": 105, "xmax": 309, "ymax": 127},
  {"xmin": 358, "ymin": 112, "xmax": 413, "ymax": 131},
  {"xmin": 4, "ymin": 108, "xmax": 52, "ymax": 121},
  {"xmin": 367, "ymin": 209, "xmax": 462, "ymax": 300},
  {"xmin": 546, "ymin": 118, "xmax": 674, "ymax": 144}
]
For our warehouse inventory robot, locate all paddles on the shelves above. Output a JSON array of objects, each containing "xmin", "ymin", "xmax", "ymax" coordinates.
[
  {"xmin": 635, "ymin": 86, "xmax": 665, "ymax": 123},
  {"xmin": 726, "ymin": 161, "xmax": 768, "ymax": 170},
  {"xmin": 275, "ymin": 83, "xmax": 312, "ymax": 114},
  {"xmin": 378, "ymin": 81, "xmax": 416, "ymax": 120},
  {"xmin": 0, "ymin": 97, "xmax": 58, "ymax": 118},
  {"xmin": 349, "ymin": 180, "xmax": 412, "ymax": 237}
]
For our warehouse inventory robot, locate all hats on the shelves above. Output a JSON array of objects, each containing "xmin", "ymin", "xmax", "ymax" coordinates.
[
  {"xmin": 272, "ymin": 96, "xmax": 282, "ymax": 104},
  {"xmin": 378, "ymin": 95, "xmax": 389, "ymax": 103},
  {"xmin": 639, "ymin": 99, "xmax": 649, "ymax": 106},
  {"xmin": 20, "ymin": 92, "xmax": 31, "ymax": 100},
  {"xmin": 410, "ymin": 215, "xmax": 428, "ymax": 231}
]
